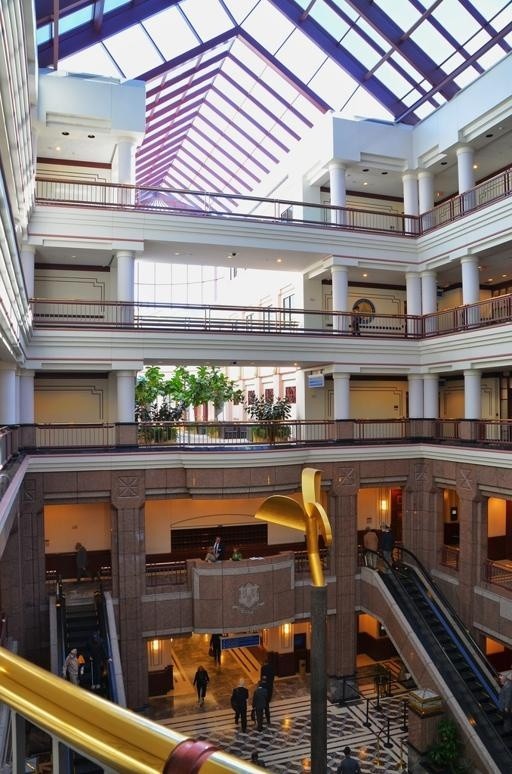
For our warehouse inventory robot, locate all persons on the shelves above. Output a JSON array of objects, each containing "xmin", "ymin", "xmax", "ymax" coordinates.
[
  {"xmin": 190, "ymin": 664, "xmax": 209, "ymax": 707},
  {"xmin": 336, "ymin": 746, "xmax": 361, "ymax": 774},
  {"xmin": 260, "ymin": 657, "xmax": 274, "ymax": 702},
  {"xmin": 497, "ymin": 674, "xmax": 511, "ymax": 735},
  {"xmin": 230, "ymin": 677, "xmax": 249, "ymax": 733},
  {"xmin": 350, "ymin": 305, "xmax": 365, "ymax": 335},
  {"xmin": 379, "ymin": 528, "xmax": 395, "ymax": 574},
  {"xmin": 62, "ymin": 627, "xmax": 112, "ymax": 694},
  {"xmin": 74, "ymin": 542, "xmax": 87, "ymax": 583},
  {"xmin": 251, "ymin": 680, "xmax": 267, "ymax": 731},
  {"xmin": 210, "ymin": 537, "xmax": 224, "ymax": 561},
  {"xmin": 247, "ymin": 751, "xmax": 265, "ymax": 768},
  {"xmin": 363, "ymin": 525, "xmax": 380, "ymax": 571},
  {"xmin": 210, "ymin": 633, "xmax": 223, "ymax": 665},
  {"xmin": 229, "ymin": 546, "xmax": 242, "ymax": 561},
  {"xmin": 204, "ymin": 545, "xmax": 218, "ymax": 564},
  {"xmin": 259, "ymin": 674, "xmax": 272, "ymax": 724}
]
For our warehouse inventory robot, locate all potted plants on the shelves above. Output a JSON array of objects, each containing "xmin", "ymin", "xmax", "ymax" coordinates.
[
  {"xmin": 134, "ymin": 364, "xmax": 245, "ymax": 446},
  {"xmin": 245, "ymin": 391, "xmax": 294, "ymax": 441}
]
